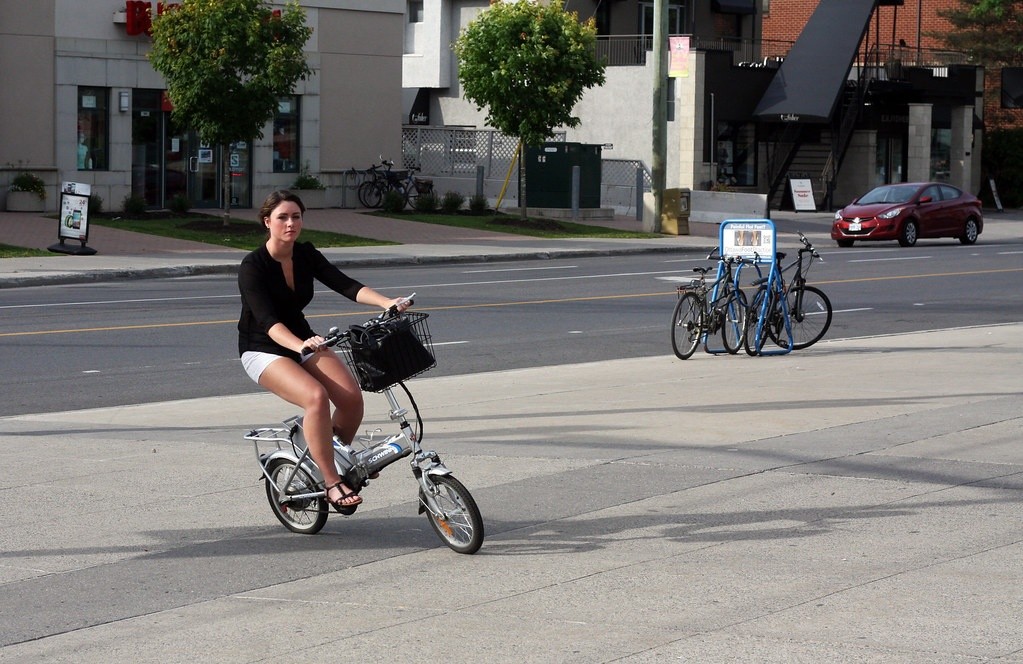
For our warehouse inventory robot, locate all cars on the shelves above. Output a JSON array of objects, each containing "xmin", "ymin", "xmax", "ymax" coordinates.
[{"xmin": 831, "ymin": 182, "xmax": 984, "ymax": 247}]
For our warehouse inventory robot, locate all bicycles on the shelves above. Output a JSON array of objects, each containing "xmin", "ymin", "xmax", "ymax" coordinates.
[
  {"xmin": 742, "ymin": 231, "xmax": 832, "ymax": 356},
  {"xmin": 357, "ymin": 164, "xmax": 408, "ymax": 210},
  {"xmin": 243, "ymin": 291, "xmax": 486, "ymax": 555},
  {"xmin": 670, "ymin": 248, "xmax": 761, "ymax": 361},
  {"xmin": 381, "ymin": 161, "xmax": 433, "ymax": 209}
]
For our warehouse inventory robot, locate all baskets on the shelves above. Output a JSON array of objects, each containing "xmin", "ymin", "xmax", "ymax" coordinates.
[{"xmin": 336, "ymin": 311, "xmax": 438, "ymax": 394}]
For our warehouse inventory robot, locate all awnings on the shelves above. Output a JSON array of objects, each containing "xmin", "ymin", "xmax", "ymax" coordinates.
[{"xmin": 161, "ymin": 91, "xmax": 175, "ymax": 110}]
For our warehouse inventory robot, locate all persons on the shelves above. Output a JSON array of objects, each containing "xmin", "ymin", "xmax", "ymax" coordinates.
[
  {"xmin": 237, "ymin": 187, "xmax": 414, "ymax": 506},
  {"xmin": 275, "ymin": 127, "xmax": 290, "ymax": 173},
  {"xmin": 77, "ymin": 132, "xmax": 93, "ymax": 169}
]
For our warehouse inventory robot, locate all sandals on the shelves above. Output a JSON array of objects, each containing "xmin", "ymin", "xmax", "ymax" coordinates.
[
  {"xmin": 369, "ymin": 472, "xmax": 379, "ymax": 479},
  {"xmin": 323, "ymin": 481, "xmax": 363, "ymax": 506}
]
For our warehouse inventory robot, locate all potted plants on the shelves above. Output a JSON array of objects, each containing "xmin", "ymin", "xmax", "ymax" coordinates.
[
  {"xmin": 5, "ymin": 172, "xmax": 48, "ymax": 212},
  {"xmin": 287, "ymin": 173, "xmax": 326, "ymax": 209}
]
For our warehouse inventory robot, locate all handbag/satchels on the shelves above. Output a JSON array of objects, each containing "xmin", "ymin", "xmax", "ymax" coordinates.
[{"xmin": 347, "ymin": 313, "xmax": 436, "ymax": 392}]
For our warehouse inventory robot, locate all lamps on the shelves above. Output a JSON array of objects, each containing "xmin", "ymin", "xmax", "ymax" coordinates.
[{"xmin": 119, "ymin": 91, "xmax": 129, "ymax": 112}]
